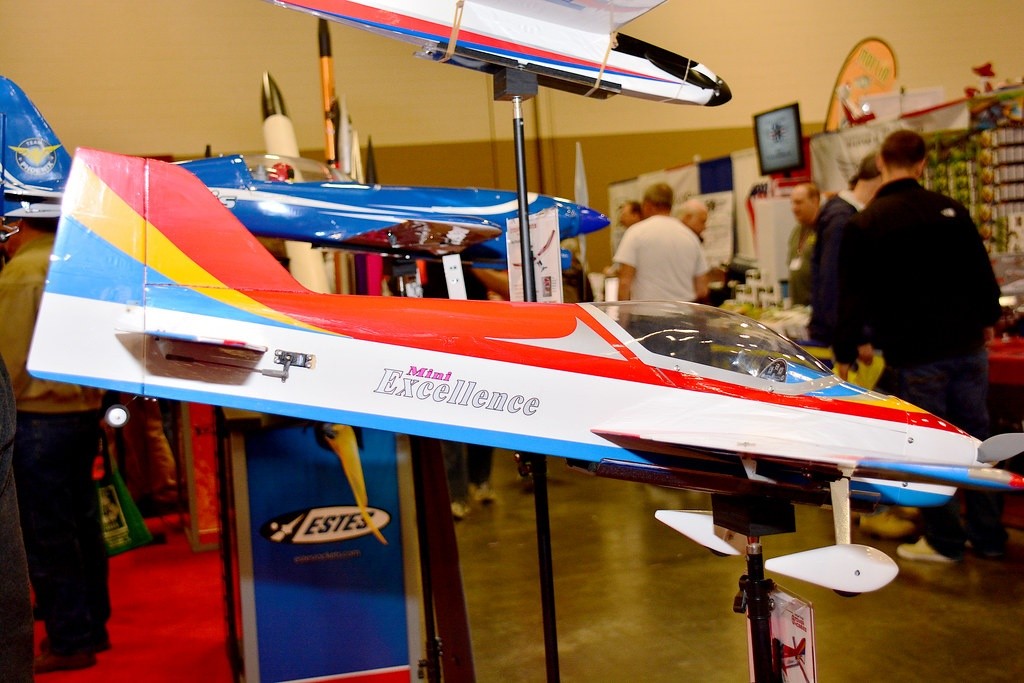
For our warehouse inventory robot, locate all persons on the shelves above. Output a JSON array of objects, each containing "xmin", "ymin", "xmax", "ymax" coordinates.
[
  {"xmin": 0, "ymin": 215, "xmax": 112, "ymax": 683},
  {"xmin": 116, "ymin": 394, "xmax": 181, "ymax": 519},
  {"xmin": 380, "ymin": 131, "xmax": 1010, "ymax": 563}
]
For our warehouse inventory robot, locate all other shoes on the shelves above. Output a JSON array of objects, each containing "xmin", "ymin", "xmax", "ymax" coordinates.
[
  {"xmin": 40, "ymin": 637, "xmax": 110, "ymax": 653},
  {"xmin": 468, "ymin": 483, "xmax": 497, "ymax": 502},
  {"xmin": 451, "ymin": 502, "xmax": 471, "ymax": 518},
  {"xmin": 964, "ymin": 539, "xmax": 1006, "ymax": 557},
  {"xmin": 897, "ymin": 536, "xmax": 961, "ymax": 563},
  {"xmin": 34, "ymin": 652, "xmax": 96, "ymax": 672}
]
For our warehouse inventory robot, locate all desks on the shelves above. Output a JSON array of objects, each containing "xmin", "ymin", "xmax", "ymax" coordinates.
[{"xmin": 706, "ymin": 338, "xmax": 838, "ymax": 376}]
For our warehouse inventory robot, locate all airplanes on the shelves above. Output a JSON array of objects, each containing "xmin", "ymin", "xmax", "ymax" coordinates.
[
  {"xmin": 263, "ymin": 0, "xmax": 732, "ymax": 108},
  {"xmin": 0, "ymin": 73, "xmax": 611, "ymax": 259},
  {"xmin": 25, "ymin": 146, "xmax": 1024, "ymax": 597}
]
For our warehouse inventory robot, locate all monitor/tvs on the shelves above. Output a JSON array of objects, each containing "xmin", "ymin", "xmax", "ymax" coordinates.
[{"xmin": 750, "ymin": 100, "xmax": 805, "ymax": 177}]
